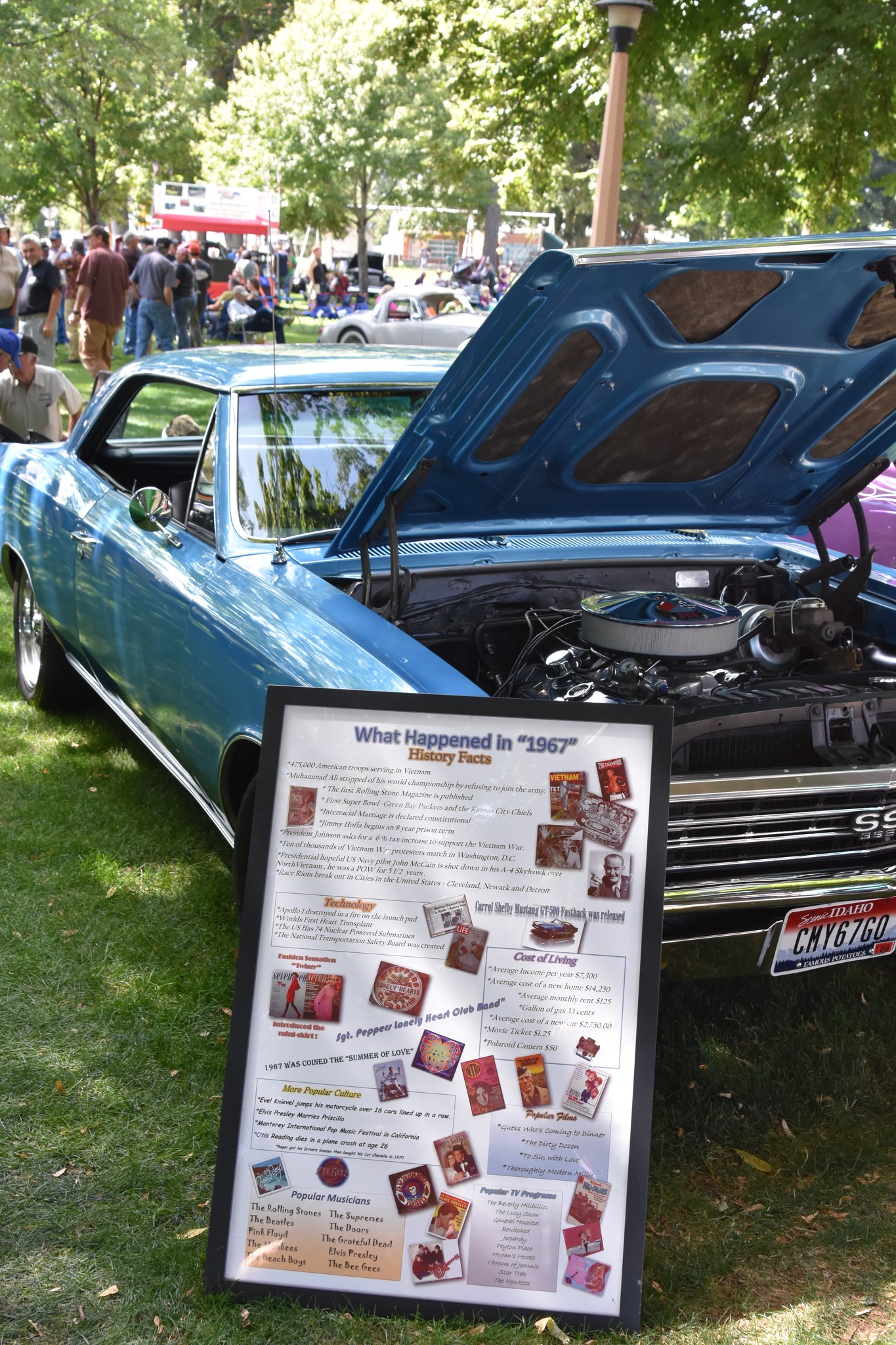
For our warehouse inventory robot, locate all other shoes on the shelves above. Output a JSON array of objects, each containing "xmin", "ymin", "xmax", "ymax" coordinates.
[
  {"xmin": 285, "ymin": 316, "xmax": 295, "ymax": 326},
  {"xmin": 61, "ymin": 359, "xmax": 81, "ymax": 363}
]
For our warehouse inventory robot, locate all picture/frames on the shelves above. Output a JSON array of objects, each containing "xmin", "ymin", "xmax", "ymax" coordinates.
[{"xmin": 199, "ymin": 681, "xmax": 677, "ymax": 1338}]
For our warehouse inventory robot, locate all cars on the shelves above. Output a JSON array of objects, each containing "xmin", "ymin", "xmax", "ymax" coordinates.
[
  {"xmin": 319, "ymin": 284, "xmax": 486, "ymax": 350},
  {"xmin": 326, "ymin": 248, "xmax": 383, "ymax": 298},
  {"xmin": 197, "ymin": 239, "xmax": 238, "ymax": 283},
  {"xmin": 0, "ymin": 227, "xmax": 895, "ymax": 999}
]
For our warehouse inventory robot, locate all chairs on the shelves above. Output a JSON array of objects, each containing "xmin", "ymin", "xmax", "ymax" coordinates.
[{"xmin": 217, "ymin": 272, "xmax": 373, "ymax": 346}]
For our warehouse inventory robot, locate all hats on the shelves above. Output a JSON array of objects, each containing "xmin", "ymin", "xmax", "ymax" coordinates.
[
  {"xmin": 46, "ymin": 231, "xmax": 61, "ymax": 240},
  {"xmin": 233, "ymin": 285, "xmax": 249, "ymax": 296},
  {"xmin": 16, "ymin": 332, "xmax": 39, "ymax": 355},
  {"xmin": 70, "ymin": 238, "xmax": 85, "ymax": 251},
  {"xmin": 41, "ymin": 240, "xmax": 49, "ymax": 251},
  {"xmin": 0, "ymin": 328, "xmax": 22, "ymax": 369},
  {"xmin": 188, "ymin": 242, "xmax": 200, "ymax": 253},
  {"xmin": 82, "ymin": 225, "xmax": 110, "ymax": 239},
  {"xmin": 156, "ymin": 237, "xmax": 170, "ymax": 248}
]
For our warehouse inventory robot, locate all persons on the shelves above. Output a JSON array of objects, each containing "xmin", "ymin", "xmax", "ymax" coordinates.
[{"xmin": 0, "ymin": 225, "xmax": 509, "ymax": 444}]
